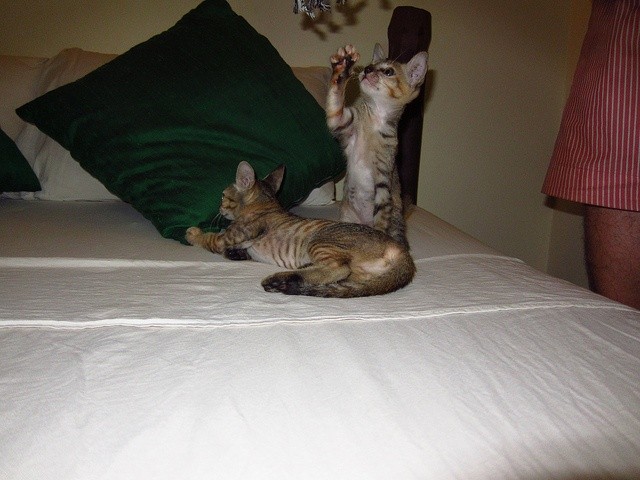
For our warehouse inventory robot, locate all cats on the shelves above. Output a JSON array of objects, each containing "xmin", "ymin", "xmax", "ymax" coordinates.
[
  {"xmin": 186, "ymin": 162, "xmax": 415, "ymax": 298},
  {"xmin": 325, "ymin": 44, "xmax": 429, "ymax": 251}
]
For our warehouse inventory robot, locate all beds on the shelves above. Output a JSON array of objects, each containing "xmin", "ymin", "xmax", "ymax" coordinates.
[{"xmin": 1, "ymin": 6, "xmax": 640, "ymax": 479}]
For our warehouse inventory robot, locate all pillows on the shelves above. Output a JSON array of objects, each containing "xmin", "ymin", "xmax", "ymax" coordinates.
[
  {"xmin": 13, "ymin": 1, "xmax": 348, "ymax": 247},
  {"xmin": 16, "ymin": 45, "xmax": 338, "ymax": 209},
  {"xmin": 0, "ymin": 124, "xmax": 44, "ymax": 197}
]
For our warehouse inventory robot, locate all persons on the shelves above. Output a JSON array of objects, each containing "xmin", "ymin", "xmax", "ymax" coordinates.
[{"xmin": 542, "ymin": 0, "xmax": 640, "ymax": 316}]
